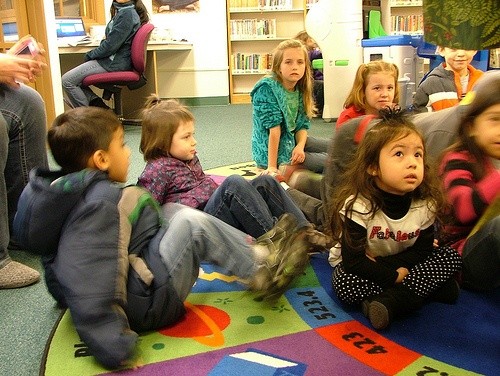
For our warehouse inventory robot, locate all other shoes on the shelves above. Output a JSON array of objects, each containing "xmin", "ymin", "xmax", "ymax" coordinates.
[
  {"xmin": 362, "ymin": 294, "xmax": 400, "ymax": 331},
  {"xmin": 428, "ymin": 277, "xmax": 462, "ymax": 304},
  {"xmin": 249, "ymin": 225, "xmax": 309, "ymax": 301},
  {"xmin": 0, "ymin": 260, "xmax": 41, "ymax": 290},
  {"xmin": 281, "ymin": 163, "xmax": 302, "ymax": 182},
  {"xmin": 255, "ymin": 213, "xmax": 300, "ymax": 290},
  {"xmin": 261, "ymin": 170, "xmax": 282, "ymax": 182}
]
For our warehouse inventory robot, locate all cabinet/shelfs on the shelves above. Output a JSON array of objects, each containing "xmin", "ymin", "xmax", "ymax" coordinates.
[
  {"xmin": 228, "ymin": 1, "xmax": 307, "ymax": 105},
  {"xmin": 364, "ymin": 0, "xmax": 500, "ymax": 70}
]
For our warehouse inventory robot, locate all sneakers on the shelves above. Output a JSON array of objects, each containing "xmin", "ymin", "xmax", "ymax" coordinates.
[{"xmin": 303, "ymin": 228, "xmax": 340, "ymax": 256}]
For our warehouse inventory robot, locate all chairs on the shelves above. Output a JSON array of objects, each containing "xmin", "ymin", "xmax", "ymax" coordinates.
[{"xmin": 81, "ymin": 22, "xmax": 155, "ymax": 128}]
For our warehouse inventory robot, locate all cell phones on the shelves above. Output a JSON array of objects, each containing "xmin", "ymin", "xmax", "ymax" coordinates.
[{"xmin": 14, "ymin": 37, "xmax": 40, "ymax": 55}]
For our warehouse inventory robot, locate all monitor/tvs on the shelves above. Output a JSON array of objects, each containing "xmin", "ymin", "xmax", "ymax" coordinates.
[
  {"xmin": 2, "ymin": 22, "xmax": 20, "ymax": 43},
  {"xmin": 55, "ymin": 17, "xmax": 87, "ymax": 48}
]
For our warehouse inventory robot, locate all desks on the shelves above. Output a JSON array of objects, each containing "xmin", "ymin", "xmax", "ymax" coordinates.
[{"xmin": 59, "ymin": 42, "xmax": 196, "ymax": 110}]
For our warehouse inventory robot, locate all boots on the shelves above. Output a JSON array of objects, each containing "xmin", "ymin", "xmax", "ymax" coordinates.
[{"xmin": 88, "ymin": 97, "xmax": 121, "ymax": 117}]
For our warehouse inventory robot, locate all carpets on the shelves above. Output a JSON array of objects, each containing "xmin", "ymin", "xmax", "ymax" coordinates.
[{"xmin": 40, "ymin": 158, "xmax": 500, "ymax": 376}]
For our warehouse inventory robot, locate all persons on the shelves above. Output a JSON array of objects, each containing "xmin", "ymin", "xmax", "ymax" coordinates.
[
  {"xmin": 135, "ymin": 93, "xmax": 314, "ymax": 239},
  {"xmin": 0, "ymin": 34, "xmax": 47, "ymax": 289},
  {"xmin": 250, "ymin": 37, "xmax": 333, "ymax": 179},
  {"xmin": 62, "ymin": 0, "xmax": 148, "ymax": 115},
  {"xmin": 327, "ymin": 58, "xmax": 399, "ymax": 172},
  {"xmin": 8, "ymin": 106, "xmax": 314, "ymax": 372},
  {"xmin": 410, "ymin": 46, "xmax": 485, "ymax": 116},
  {"xmin": 430, "ymin": 76, "xmax": 500, "ymax": 308},
  {"xmin": 329, "ymin": 105, "xmax": 463, "ymax": 332},
  {"xmin": 256, "ymin": 102, "xmax": 471, "ymax": 239},
  {"xmin": 293, "ymin": 29, "xmax": 323, "ymax": 81}
]
